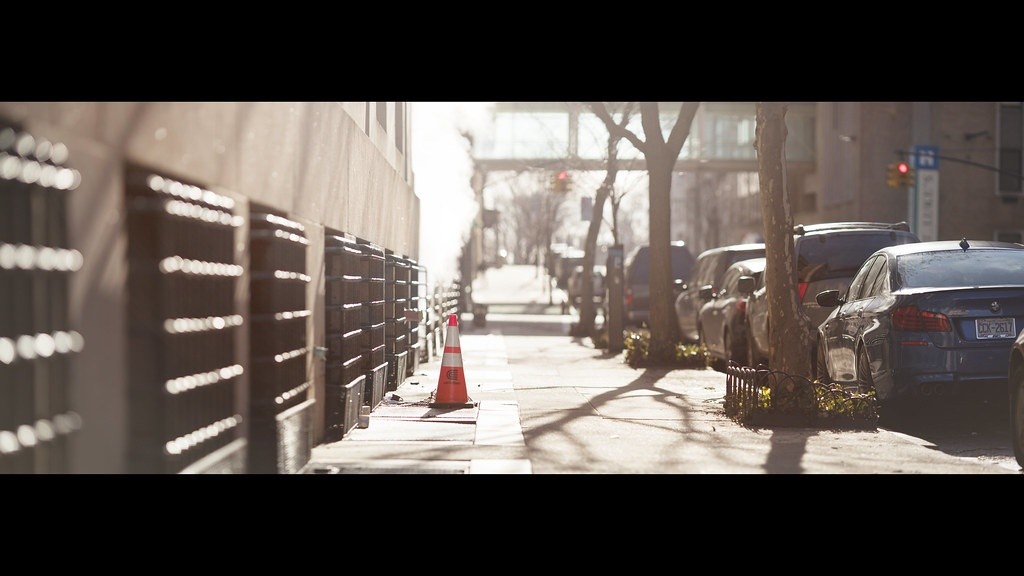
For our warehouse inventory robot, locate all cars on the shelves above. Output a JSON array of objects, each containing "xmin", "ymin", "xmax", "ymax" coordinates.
[
  {"xmin": 569, "ymin": 263, "xmax": 608, "ymax": 301},
  {"xmin": 698, "ymin": 254, "xmax": 766, "ymax": 371},
  {"xmin": 814, "ymin": 236, "xmax": 1024, "ymax": 424}
]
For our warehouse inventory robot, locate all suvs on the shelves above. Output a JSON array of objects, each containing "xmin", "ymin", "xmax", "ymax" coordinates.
[
  {"xmin": 671, "ymin": 242, "xmax": 768, "ymax": 350},
  {"xmin": 619, "ymin": 239, "xmax": 696, "ymax": 326},
  {"xmin": 736, "ymin": 220, "xmax": 928, "ymax": 375}
]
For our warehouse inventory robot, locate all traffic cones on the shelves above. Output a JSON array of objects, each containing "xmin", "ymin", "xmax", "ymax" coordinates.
[{"xmin": 427, "ymin": 313, "xmax": 478, "ymax": 408}]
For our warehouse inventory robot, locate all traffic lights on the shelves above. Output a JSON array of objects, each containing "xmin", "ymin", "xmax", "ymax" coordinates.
[
  {"xmin": 899, "ymin": 162, "xmax": 908, "ymax": 174},
  {"xmin": 886, "ymin": 163, "xmax": 900, "ymax": 187},
  {"xmin": 906, "ymin": 163, "xmax": 915, "ymax": 187},
  {"xmin": 550, "ymin": 170, "xmax": 573, "ymax": 193}
]
